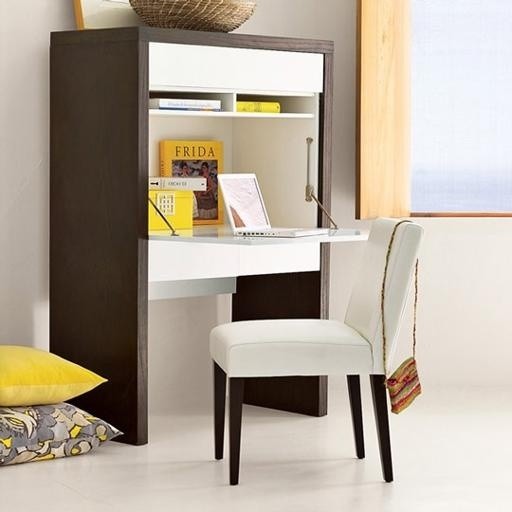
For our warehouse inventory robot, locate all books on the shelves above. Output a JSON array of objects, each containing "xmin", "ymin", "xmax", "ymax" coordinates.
[
  {"xmin": 236, "ymin": 102, "xmax": 281, "ymax": 114},
  {"xmin": 147, "ymin": 98, "xmax": 226, "ymax": 112}
]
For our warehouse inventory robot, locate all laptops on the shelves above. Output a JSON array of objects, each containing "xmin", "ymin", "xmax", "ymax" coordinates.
[{"xmin": 217, "ymin": 172, "xmax": 329, "ymax": 238}]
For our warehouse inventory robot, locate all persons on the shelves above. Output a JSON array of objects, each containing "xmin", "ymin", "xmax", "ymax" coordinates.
[
  {"xmin": 175, "ymin": 162, "xmax": 200, "ymax": 219},
  {"xmin": 193, "ymin": 160, "xmax": 217, "ymax": 220}
]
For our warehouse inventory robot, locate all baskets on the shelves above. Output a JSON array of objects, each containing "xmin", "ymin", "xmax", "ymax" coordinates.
[{"xmin": 129, "ymin": 0, "xmax": 257, "ymax": 33}]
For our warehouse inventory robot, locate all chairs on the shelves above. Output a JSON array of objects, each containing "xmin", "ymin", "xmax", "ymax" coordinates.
[{"xmin": 208, "ymin": 216, "xmax": 426, "ymax": 486}]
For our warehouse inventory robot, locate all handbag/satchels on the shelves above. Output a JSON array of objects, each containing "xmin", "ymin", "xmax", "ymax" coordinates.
[{"xmin": 387, "ymin": 358, "xmax": 421, "ymax": 414}]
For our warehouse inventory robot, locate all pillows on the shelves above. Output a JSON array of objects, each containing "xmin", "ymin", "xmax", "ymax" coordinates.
[{"xmin": 1, "ymin": 343, "xmax": 126, "ymax": 468}]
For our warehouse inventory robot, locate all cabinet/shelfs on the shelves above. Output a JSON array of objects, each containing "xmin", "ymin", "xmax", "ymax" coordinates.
[{"xmin": 49, "ymin": 26, "xmax": 336, "ymax": 446}]
